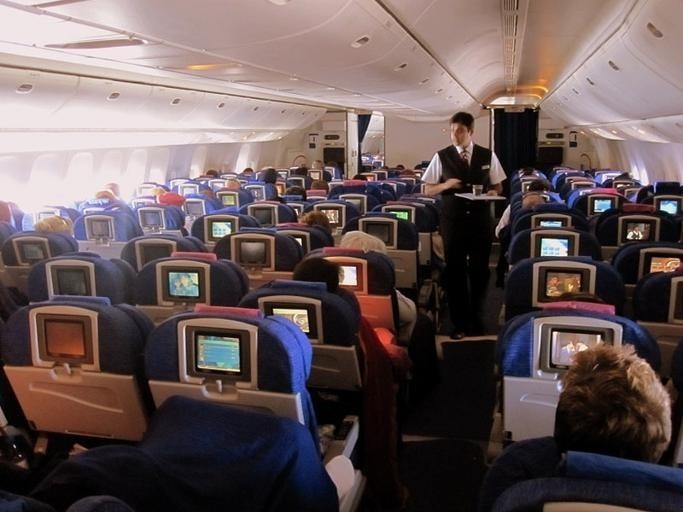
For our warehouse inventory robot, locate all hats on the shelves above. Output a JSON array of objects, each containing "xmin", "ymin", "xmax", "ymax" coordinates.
[
  {"xmin": 32, "ymin": 215, "xmax": 74, "ymax": 236},
  {"xmin": 157, "ymin": 192, "xmax": 186, "ymax": 207}
]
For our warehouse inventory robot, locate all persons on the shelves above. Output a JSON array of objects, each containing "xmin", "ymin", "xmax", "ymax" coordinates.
[
  {"xmin": 567, "ymin": 279, "xmax": 581, "ymax": 294},
  {"xmin": 0, "ymin": 153, "xmax": 419, "ymax": 236},
  {"xmin": 291, "ymin": 257, "xmax": 410, "ymax": 374},
  {"xmin": 340, "ymin": 231, "xmax": 442, "ymax": 389},
  {"xmin": 546, "ymin": 275, "xmax": 564, "ymax": 297},
  {"xmin": 494, "ymin": 179, "xmax": 553, "ymax": 279},
  {"xmin": 559, "ymin": 336, "xmax": 576, "ymax": 363},
  {"xmin": 498, "ymin": 191, "xmax": 547, "ymax": 247},
  {"xmin": 571, "ymin": 338, "xmax": 588, "ymax": 354},
  {"xmin": 552, "ymin": 340, "xmax": 676, "ymax": 491},
  {"xmin": 625, "ymin": 225, "xmax": 644, "ymax": 241},
  {"xmin": 419, "ymin": 111, "xmax": 508, "ymax": 340}
]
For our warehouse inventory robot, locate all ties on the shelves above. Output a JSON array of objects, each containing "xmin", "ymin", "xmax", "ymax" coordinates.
[{"xmin": 463, "ymin": 151, "xmax": 470, "ymax": 169}]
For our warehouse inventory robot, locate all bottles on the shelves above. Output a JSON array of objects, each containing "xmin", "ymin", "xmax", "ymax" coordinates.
[{"xmin": 7, "ymin": 439, "xmax": 29, "ymax": 471}]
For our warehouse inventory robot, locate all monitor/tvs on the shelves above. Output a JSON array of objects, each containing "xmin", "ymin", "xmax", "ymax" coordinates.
[
  {"xmin": 540, "ymin": 324, "xmax": 613, "ymax": 374},
  {"xmin": 574, "ymin": 184, "xmax": 593, "ymax": 189},
  {"xmin": 535, "ymin": 234, "xmax": 574, "ymax": 257},
  {"xmin": 219, "ymin": 194, "xmax": 237, "ymax": 207},
  {"xmin": 139, "ymin": 210, "xmax": 163, "ymax": 228},
  {"xmin": 645, "ymin": 253, "xmax": 682, "ymax": 274},
  {"xmin": 86, "ymin": 218, "xmax": 113, "ymax": 239},
  {"xmin": 365, "ymin": 175, "xmax": 375, "ymax": 181},
  {"xmin": 607, "ymin": 176, "xmax": 615, "ymax": 180},
  {"xmin": 288, "ymin": 180, "xmax": 302, "ymax": 189},
  {"xmin": 38, "ymin": 212, "xmax": 55, "ymax": 219},
  {"xmin": 310, "ymin": 171, "xmax": 320, "ymax": 181},
  {"xmin": 657, "ymin": 198, "xmax": 680, "ymax": 216},
  {"xmin": 139, "ymin": 187, "xmax": 154, "ymax": 194},
  {"xmin": 139, "ymin": 244, "xmax": 172, "ymax": 267},
  {"xmin": 249, "ymin": 188, "xmax": 263, "ymax": 200},
  {"xmin": 615, "ymin": 183, "xmax": 632, "ymax": 188},
  {"xmin": 377, "ymin": 173, "xmax": 385, "ymax": 180},
  {"xmin": 341, "ymin": 197, "xmax": 364, "ymax": 216},
  {"xmin": 250, "ymin": 207, "xmax": 275, "ymax": 227},
  {"xmin": 172, "ymin": 182, "xmax": 185, "ymax": 186},
  {"xmin": 290, "ymin": 206, "xmax": 300, "ymax": 218},
  {"xmin": 317, "ymin": 207, "xmax": 342, "ymax": 227},
  {"xmin": 212, "ymin": 183, "xmax": 225, "ymax": 191},
  {"xmin": 276, "ymin": 184, "xmax": 284, "ymax": 195},
  {"xmin": 385, "ymin": 208, "xmax": 411, "ymax": 223},
  {"xmin": 186, "ymin": 326, "xmax": 251, "ymax": 382},
  {"xmin": 590, "ymin": 197, "xmax": 614, "ymax": 215},
  {"xmin": 222, "ymin": 176, "xmax": 234, "ymax": 180},
  {"xmin": 278, "ymin": 171, "xmax": 287, "ymax": 179},
  {"xmin": 182, "ymin": 187, "xmax": 197, "ymax": 194},
  {"xmin": 621, "ymin": 219, "xmax": 656, "ymax": 243},
  {"xmin": 161, "ymin": 266, "xmax": 206, "ymax": 303},
  {"xmin": 290, "ymin": 235, "xmax": 307, "ymax": 254},
  {"xmin": 375, "ymin": 162, "xmax": 381, "ymax": 166},
  {"xmin": 332, "ymin": 262, "xmax": 363, "ymax": 292},
  {"xmin": 208, "ymin": 219, "xmax": 234, "ymax": 241},
  {"xmin": 234, "ymin": 239, "xmax": 270, "ymax": 268},
  {"xmin": 535, "ymin": 217, "xmax": 567, "ymax": 229},
  {"xmin": 415, "ymin": 172, "xmax": 422, "ymax": 178},
  {"xmin": 524, "ymin": 185, "xmax": 530, "ymax": 191},
  {"xmin": 36, "ymin": 314, "xmax": 93, "ymax": 365},
  {"xmin": 263, "ymin": 302, "xmax": 318, "ymax": 339},
  {"xmin": 51, "ymin": 265, "xmax": 91, "ymax": 296},
  {"xmin": 362, "ymin": 221, "xmax": 394, "ymax": 246},
  {"xmin": 184, "ymin": 202, "xmax": 203, "ymax": 216},
  {"xmin": 538, "ymin": 266, "xmax": 589, "ymax": 303},
  {"xmin": 16, "ymin": 241, "xmax": 48, "ymax": 263}
]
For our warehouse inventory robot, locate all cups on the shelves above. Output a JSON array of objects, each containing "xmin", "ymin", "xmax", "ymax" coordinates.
[{"xmin": 472, "ymin": 184, "xmax": 483, "ymax": 197}]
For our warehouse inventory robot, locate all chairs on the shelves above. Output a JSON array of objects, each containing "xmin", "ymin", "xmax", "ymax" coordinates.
[
  {"xmin": 339, "ymin": 213, "xmax": 433, "ymax": 310},
  {"xmin": 477, "ymin": 435, "xmax": 682, "ymax": 511},
  {"xmin": 131, "ymin": 179, "xmax": 237, "ymax": 214},
  {"xmin": 211, "ymin": 226, "xmax": 302, "ymax": 291},
  {"xmin": 190, "ymin": 215, "xmax": 262, "ymax": 254},
  {"xmin": 73, "ymin": 212, "xmax": 145, "ymax": 259},
  {"xmin": 76, "ymin": 198, "xmax": 132, "ymax": 216},
  {"xmin": 300, "ymin": 200, "xmax": 359, "ymax": 237},
  {"xmin": 567, "ymin": 189, "xmax": 631, "ymax": 226},
  {"xmin": 588, "ymin": 168, "xmax": 682, "ymax": 203},
  {"xmin": 610, "ymin": 241, "xmax": 683, "ymax": 300},
  {"xmin": 26, "ymin": 252, "xmax": 131, "ymax": 305},
  {"xmin": 237, "ymin": 280, "xmax": 374, "ymax": 405},
  {"xmin": 238, "ymin": 201, "xmax": 297, "ymax": 228},
  {"xmin": 271, "ymin": 222, "xmax": 335, "ymax": 261},
  {"xmin": 143, "ymin": 305, "xmax": 359, "ymax": 462},
  {"xmin": 2, "ymin": 230, "xmax": 78, "ymax": 294},
  {"xmin": 507, "ymin": 227, "xmax": 602, "ymax": 270},
  {"xmin": 284, "ymin": 201, "xmax": 311, "ymax": 216},
  {"xmin": 510, "ymin": 200, "xmax": 567, "ymax": 205},
  {"xmin": 511, "ymin": 206, "xmax": 521, "ymax": 220},
  {"xmin": 510, "ymin": 205, "xmax": 591, "ymax": 229},
  {"xmin": 346, "ymin": 200, "xmax": 359, "ymax": 212},
  {"xmin": 368, "ymin": 201, "xmax": 431, "ymax": 271},
  {"xmin": 632, "ymin": 270, "xmax": 683, "ymax": 384},
  {"xmin": 504, "ymin": 257, "xmax": 627, "ymax": 322},
  {"xmin": 511, "ymin": 167, "xmax": 605, "ymax": 200},
  {"xmin": 0, "ymin": 200, "xmax": 73, "ymax": 237},
  {"xmin": 0, "ymin": 298, "xmax": 146, "ymax": 445},
  {"xmin": 360, "ymin": 201, "xmax": 368, "ymax": 212},
  {"xmin": 133, "ymin": 206, "xmax": 189, "ymax": 238},
  {"xmin": 120, "ymin": 231, "xmax": 182, "ymax": 277},
  {"xmin": 369, "ymin": 201, "xmax": 386, "ymax": 212},
  {"xmin": 302, "ymin": 248, "xmax": 405, "ymax": 352},
  {"xmin": 126, "ymin": 394, "xmax": 363, "ymax": 511},
  {"xmin": 639, "ymin": 190, "xmax": 683, "ymax": 220},
  {"xmin": 131, "ymin": 253, "xmax": 250, "ymax": 326},
  {"xmin": 596, "ymin": 203, "xmax": 679, "ymax": 263},
  {"xmin": 166, "ymin": 154, "xmax": 436, "ymax": 200},
  {"xmin": 489, "ymin": 301, "xmax": 663, "ymax": 450}
]
